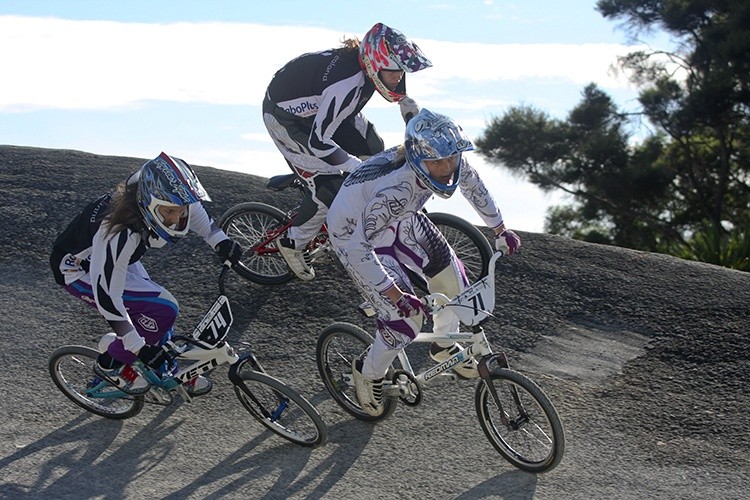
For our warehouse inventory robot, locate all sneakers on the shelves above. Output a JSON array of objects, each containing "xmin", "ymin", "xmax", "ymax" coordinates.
[
  {"xmin": 428, "ymin": 341, "xmax": 480, "ymax": 380},
  {"xmin": 276, "ymin": 230, "xmax": 315, "ymax": 282},
  {"xmin": 92, "ymin": 352, "xmax": 152, "ymax": 397},
  {"xmin": 183, "ymin": 374, "xmax": 213, "ymax": 397},
  {"xmin": 351, "ymin": 357, "xmax": 386, "ymax": 417}
]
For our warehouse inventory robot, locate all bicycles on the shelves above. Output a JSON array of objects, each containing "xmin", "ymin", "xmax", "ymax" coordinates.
[
  {"xmin": 48, "ymin": 254, "xmax": 328, "ymax": 450},
  {"xmin": 217, "ymin": 114, "xmax": 493, "ymax": 303},
  {"xmin": 314, "ymin": 246, "xmax": 566, "ymax": 474}
]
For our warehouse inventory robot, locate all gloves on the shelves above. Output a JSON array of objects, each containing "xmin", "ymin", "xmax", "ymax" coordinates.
[
  {"xmin": 396, "ymin": 95, "xmax": 420, "ymax": 123},
  {"xmin": 213, "ymin": 239, "xmax": 244, "ymax": 268},
  {"xmin": 394, "ymin": 292, "xmax": 431, "ymax": 322},
  {"xmin": 137, "ymin": 344, "xmax": 172, "ymax": 370},
  {"xmin": 493, "ymin": 227, "xmax": 522, "ymax": 257}
]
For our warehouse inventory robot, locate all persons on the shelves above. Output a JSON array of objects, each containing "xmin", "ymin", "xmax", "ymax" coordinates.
[
  {"xmin": 49, "ymin": 151, "xmax": 240, "ymax": 394},
  {"xmin": 325, "ymin": 105, "xmax": 521, "ymax": 416},
  {"xmin": 262, "ymin": 22, "xmax": 436, "ymax": 282}
]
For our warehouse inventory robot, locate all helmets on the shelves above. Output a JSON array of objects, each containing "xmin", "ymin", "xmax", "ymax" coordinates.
[
  {"xmin": 402, "ymin": 107, "xmax": 477, "ymax": 200},
  {"xmin": 356, "ymin": 22, "xmax": 433, "ymax": 104},
  {"xmin": 136, "ymin": 152, "xmax": 215, "ymax": 246}
]
